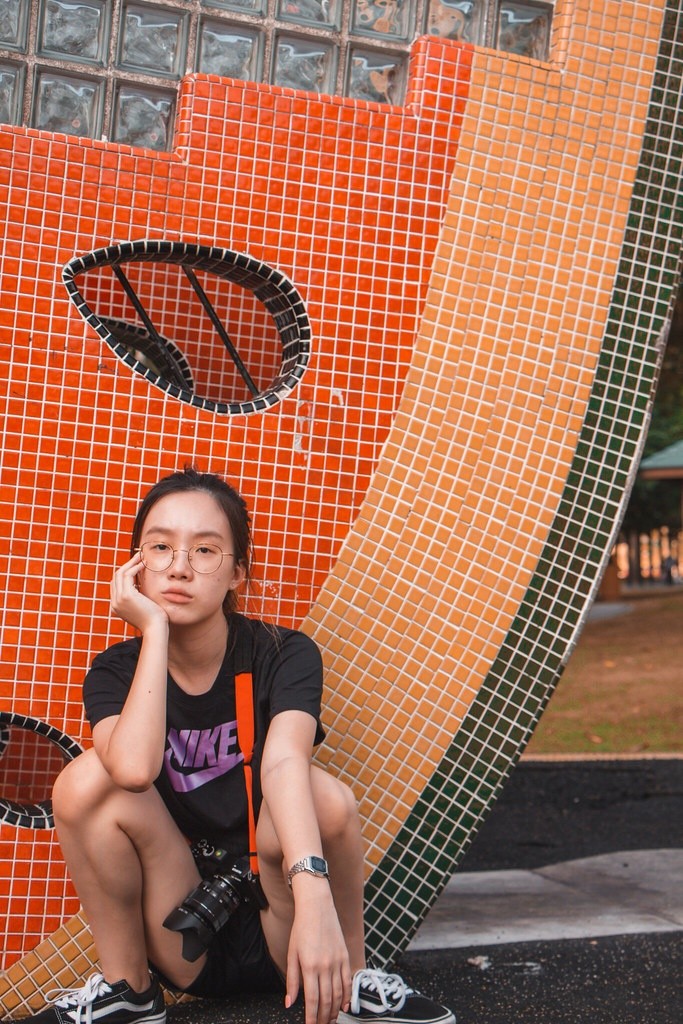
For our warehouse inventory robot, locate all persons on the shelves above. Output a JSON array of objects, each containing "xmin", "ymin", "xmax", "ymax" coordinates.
[{"xmin": 24, "ymin": 472, "xmax": 459, "ymax": 1024}]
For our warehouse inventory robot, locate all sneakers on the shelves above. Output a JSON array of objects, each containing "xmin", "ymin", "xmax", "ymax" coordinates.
[
  {"xmin": 12, "ymin": 971, "xmax": 167, "ymax": 1024},
  {"xmin": 335, "ymin": 967, "xmax": 456, "ymax": 1024}
]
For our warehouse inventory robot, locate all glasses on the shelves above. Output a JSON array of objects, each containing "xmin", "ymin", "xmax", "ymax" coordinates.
[{"xmin": 134, "ymin": 540, "xmax": 235, "ymax": 574}]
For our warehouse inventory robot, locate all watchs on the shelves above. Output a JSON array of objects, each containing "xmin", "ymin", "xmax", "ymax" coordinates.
[{"xmin": 288, "ymin": 855, "xmax": 331, "ymax": 888}]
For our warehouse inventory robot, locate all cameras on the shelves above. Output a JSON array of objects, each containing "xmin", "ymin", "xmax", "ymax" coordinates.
[{"xmin": 161, "ymin": 838, "xmax": 270, "ymax": 964}]
